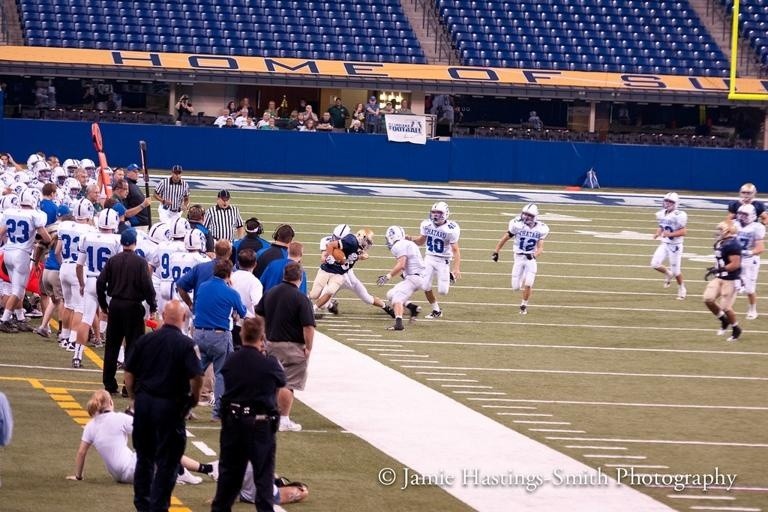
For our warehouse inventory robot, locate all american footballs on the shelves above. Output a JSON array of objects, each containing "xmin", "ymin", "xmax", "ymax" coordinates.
[{"xmin": 332, "ymin": 248, "xmax": 346, "ymax": 264}]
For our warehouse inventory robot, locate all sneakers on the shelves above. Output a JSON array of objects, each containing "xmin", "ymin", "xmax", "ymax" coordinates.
[
  {"xmin": 384, "ymin": 326, "xmax": 405, "ymax": 332},
  {"xmin": 409, "ymin": 305, "xmax": 421, "ymax": 323},
  {"xmin": 519, "ymin": 304, "xmax": 528, "ymax": 315},
  {"xmin": 715, "ymin": 317, "xmax": 731, "ymax": 335},
  {"xmin": 173, "ymin": 467, "xmax": 204, "ymax": 485},
  {"xmin": 24, "ymin": 307, "xmax": 43, "ymax": 318},
  {"xmin": 58, "ymin": 338, "xmax": 70, "ymax": 351},
  {"xmin": 745, "ymin": 310, "xmax": 759, "ymax": 321},
  {"xmin": 662, "ymin": 274, "xmax": 674, "ymax": 288},
  {"xmin": 207, "ymin": 459, "xmax": 219, "ymax": 482},
  {"xmin": 449, "ymin": 273, "xmax": 456, "ymax": 286},
  {"xmin": 86, "ymin": 335, "xmax": 94, "ymax": 348},
  {"xmin": 424, "ymin": 309, "xmax": 443, "ymax": 320},
  {"xmin": 71, "ymin": 356, "xmax": 82, "ymax": 369},
  {"xmin": 675, "ymin": 287, "xmax": 688, "ymax": 299},
  {"xmin": 381, "ymin": 301, "xmax": 396, "ymax": 320},
  {"xmin": 0, "ymin": 319, "xmax": 17, "ymax": 333},
  {"xmin": 328, "ymin": 301, "xmax": 339, "ymax": 315},
  {"xmin": 14, "ymin": 320, "xmax": 35, "ymax": 332},
  {"xmin": 65, "ymin": 341, "xmax": 76, "ymax": 352},
  {"xmin": 120, "ymin": 385, "xmax": 130, "ymax": 397},
  {"xmin": 727, "ymin": 327, "xmax": 742, "ymax": 341},
  {"xmin": 105, "ymin": 386, "xmax": 120, "ymax": 395},
  {"xmin": 36, "ymin": 326, "xmax": 50, "ymax": 337},
  {"xmin": 100, "ymin": 334, "xmax": 109, "ymax": 344},
  {"xmin": 277, "ymin": 418, "xmax": 304, "ymax": 433},
  {"xmin": 94, "ymin": 337, "xmax": 103, "ymax": 348}
]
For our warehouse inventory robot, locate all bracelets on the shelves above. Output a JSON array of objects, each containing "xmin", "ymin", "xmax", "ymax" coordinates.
[{"xmin": 74, "ymin": 474, "xmax": 87, "ymax": 480}]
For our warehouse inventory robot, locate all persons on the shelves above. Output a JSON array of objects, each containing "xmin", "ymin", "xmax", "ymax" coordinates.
[
  {"xmin": 648, "ymin": 192, "xmax": 688, "ymax": 301},
  {"xmin": 0, "ymin": 151, "xmax": 317, "ymax": 450},
  {"xmin": 702, "ymin": 218, "xmax": 742, "ymax": 342},
  {"xmin": 405, "ymin": 201, "xmax": 462, "ymax": 321},
  {"xmin": 492, "ymin": 202, "xmax": 550, "ymax": 317},
  {"xmin": 376, "ymin": 225, "xmax": 426, "ymax": 331},
  {"xmin": 307, "ymin": 224, "xmax": 394, "ymax": 320},
  {"xmin": 239, "ymin": 460, "xmax": 310, "ymax": 506},
  {"xmin": 174, "ymin": 86, "xmax": 460, "ymax": 135},
  {"xmin": 727, "ymin": 182, "xmax": 767, "ymax": 321},
  {"xmin": 122, "ymin": 299, "xmax": 204, "ymax": 512},
  {"xmin": 64, "ymin": 389, "xmax": 217, "ymax": 482},
  {"xmin": 211, "ymin": 315, "xmax": 289, "ymax": 512}
]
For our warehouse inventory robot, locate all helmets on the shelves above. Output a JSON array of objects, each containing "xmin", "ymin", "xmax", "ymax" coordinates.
[
  {"xmin": 167, "ymin": 215, "xmax": 189, "ymax": 239},
  {"xmin": 738, "ymin": 183, "xmax": 756, "ymax": 201},
  {"xmin": 0, "ymin": 193, "xmax": 19, "ymax": 212},
  {"xmin": 13, "ymin": 171, "xmax": 29, "ymax": 184},
  {"xmin": 332, "ymin": 223, "xmax": 351, "ymax": 241},
  {"xmin": 49, "ymin": 167, "xmax": 67, "ymax": 185},
  {"xmin": 148, "ymin": 221, "xmax": 173, "ymax": 246},
  {"xmin": 661, "ymin": 192, "xmax": 680, "ymax": 210},
  {"xmin": 186, "ymin": 229, "xmax": 207, "ymax": 253},
  {"xmin": 97, "ymin": 207, "xmax": 120, "ymax": 230},
  {"xmin": 737, "ymin": 203, "xmax": 757, "ymax": 224},
  {"xmin": 61, "ymin": 177, "xmax": 83, "ymax": 196},
  {"xmin": 72, "ymin": 198, "xmax": 95, "ymax": 222},
  {"xmin": 10, "ymin": 182, "xmax": 27, "ymax": 196},
  {"xmin": 62, "ymin": 157, "xmax": 78, "ymax": 172},
  {"xmin": 94, "ymin": 165, "xmax": 113, "ymax": 182},
  {"xmin": 384, "ymin": 225, "xmax": 406, "ymax": 247},
  {"xmin": 521, "ymin": 202, "xmax": 538, "ymax": 221},
  {"xmin": 0, "ymin": 159, "xmax": 5, "ymax": 174},
  {"xmin": 715, "ymin": 220, "xmax": 736, "ymax": 239},
  {"xmin": 429, "ymin": 202, "xmax": 450, "ymax": 223},
  {"xmin": 80, "ymin": 157, "xmax": 94, "ymax": 173},
  {"xmin": 21, "ymin": 188, "xmax": 42, "ymax": 209},
  {"xmin": 25, "ymin": 154, "xmax": 43, "ymax": 168},
  {"xmin": 32, "ymin": 160, "xmax": 51, "ymax": 177},
  {"xmin": 23, "ymin": 170, "xmax": 36, "ymax": 180},
  {"xmin": 354, "ymin": 229, "xmax": 374, "ymax": 248}
]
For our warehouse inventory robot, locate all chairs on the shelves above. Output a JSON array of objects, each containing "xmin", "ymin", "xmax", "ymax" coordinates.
[
  {"xmin": 719, "ymin": 0, "xmax": 768, "ymax": 71},
  {"xmin": 6, "ymin": 104, "xmax": 173, "ymax": 123},
  {"xmin": 432, "ymin": 0, "xmax": 731, "ymax": 77},
  {"xmin": 180, "ymin": 114, "xmax": 293, "ymax": 129}
]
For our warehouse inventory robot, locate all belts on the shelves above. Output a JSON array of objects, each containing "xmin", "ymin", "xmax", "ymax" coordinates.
[{"xmin": 194, "ymin": 327, "xmax": 230, "ymax": 333}]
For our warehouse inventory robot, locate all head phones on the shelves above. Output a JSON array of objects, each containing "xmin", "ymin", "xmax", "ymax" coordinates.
[
  {"xmin": 272, "ymin": 230, "xmax": 277, "ymax": 239},
  {"xmin": 256, "ymin": 223, "xmax": 264, "ymax": 235}
]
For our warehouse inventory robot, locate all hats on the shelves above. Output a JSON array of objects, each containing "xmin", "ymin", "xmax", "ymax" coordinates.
[
  {"xmin": 55, "ymin": 204, "xmax": 72, "ymax": 218},
  {"xmin": 243, "ymin": 218, "xmax": 263, "ymax": 236},
  {"xmin": 125, "ymin": 162, "xmax": 143, "ymax": 173},
  {"xmin": 215, "ymin": 189, "xmax": 230, "ymax": 200},
  {"xmin": 369, "ymin": 95, "xmax": 376, "ymax": 101},
  {"xmin": 170, "ymin": 165, "xmax": 184, "ymax": 174},
  {"xmin": 119, "ymin": 227, "xmax": 138, "ymax": 246}
]
